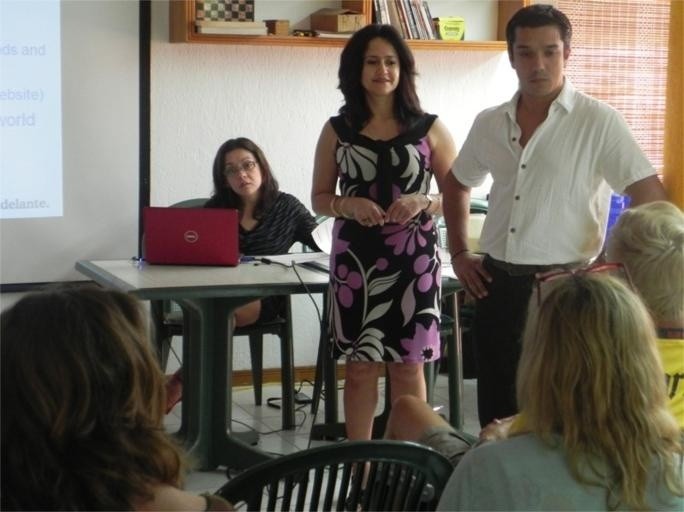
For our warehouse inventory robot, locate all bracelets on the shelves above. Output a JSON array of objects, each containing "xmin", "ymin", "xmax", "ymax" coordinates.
[
  {"xmin": 420, "ymin": 192, "xmax": 432, "ymax": 211},
  {"xmin": 339, "ymin": 195, "xmax": 356, "ymax": 222},
  {"xmin": 429, "ymin": 194, "xmax": 441, "ymax": 215},
  {"xmin": 330, "ymin": 193, "xmax": 341, "ymax": 217},
  {"xmin": 450, "ymin": 249, "xmax": 470, "ymax": 260}
]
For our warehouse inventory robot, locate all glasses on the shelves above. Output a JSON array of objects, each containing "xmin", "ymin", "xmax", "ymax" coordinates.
[
  {"xmin": 225, "ymin": 161, "xmax": 256, "ymax": 176},
  {"xmin": 534, "ymin": 262, "xmax": 636, "ymax": 307}
]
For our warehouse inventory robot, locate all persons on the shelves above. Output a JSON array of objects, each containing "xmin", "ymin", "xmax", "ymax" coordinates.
[
  {"xmin": 311, "ymin": 23, "xmax": 458, "ymax": 508},
  {"xmin": 441, "ymin": 6, "xmax": 670, "ymax": 426},
  {"xmin": 438, "ymin": 255, "xmax": 683, "ymax": 512},
  {"xmin": 156, "ymin": 136, "xmax": 318, "ymax": 413},
  {"xmin": 378, "ymin": 197, "xmax": 683, "ymax": 512},
  {"xmin": 0, "ymin": 282, "xmax": 240, "ymax": 512}
]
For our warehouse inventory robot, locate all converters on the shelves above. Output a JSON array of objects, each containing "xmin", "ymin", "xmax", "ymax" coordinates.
[{"xmin": 295, "ymin": 392, "xmax": 312, "ymax": 404}]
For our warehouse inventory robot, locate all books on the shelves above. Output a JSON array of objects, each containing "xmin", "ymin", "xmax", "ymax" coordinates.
[
  {"xmin": 301, "ymin": 215, "xmax": 335, "ymax": 273},
  {"xmin": 254, "ymin": 250, "xmax": 319, "ymax": 267},
  {"xmin": 193, "ymin": 1, "xmax": 440, "ymax": 40}
]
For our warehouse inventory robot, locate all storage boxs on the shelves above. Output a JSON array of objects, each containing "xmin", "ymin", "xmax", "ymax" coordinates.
[{"xmin": 311, "ymin": 6, "xmax": 368, "ymax": 34}]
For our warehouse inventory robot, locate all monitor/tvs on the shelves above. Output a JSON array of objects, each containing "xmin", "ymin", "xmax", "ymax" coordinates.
[{"xmin": 607, "ymin": 191, "xmax": 630, "ymax": 240}]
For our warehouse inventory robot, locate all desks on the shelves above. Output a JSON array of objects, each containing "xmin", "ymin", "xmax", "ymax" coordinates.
[{"xmin": 75, "ymin": 252, "xmax": 471, "ymax": 468}]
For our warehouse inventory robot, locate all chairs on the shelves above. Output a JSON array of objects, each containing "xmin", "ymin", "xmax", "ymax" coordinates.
[
  {"xmin": 211, "ymin": 437, "xmax": 453, "ymax": 511},
  {"xmin": 147, "ymin": 195, "xmax": 298, "ymax": 431}
]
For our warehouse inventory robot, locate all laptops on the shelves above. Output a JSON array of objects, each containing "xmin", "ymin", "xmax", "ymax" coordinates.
[{"xmin": 142, "ymin": 205, "xmax": 245, "ymax": 267}]
